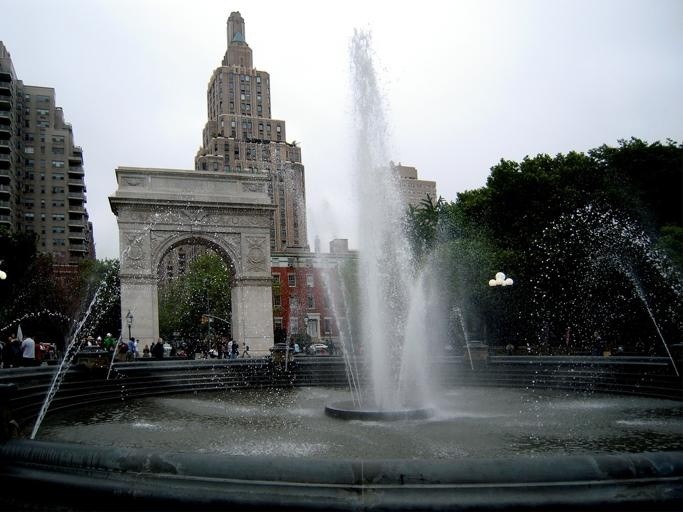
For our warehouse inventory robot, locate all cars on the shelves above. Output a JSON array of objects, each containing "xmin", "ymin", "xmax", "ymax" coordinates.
[
  {"xmin": 312, "ymin": 344, "xmax": 328, "ymax": 351},
  {"xmin": 269, "ymin": 343, "xmax": 295, "ymax": 355}
]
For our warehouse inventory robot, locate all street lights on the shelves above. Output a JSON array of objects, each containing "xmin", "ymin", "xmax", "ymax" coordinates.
[
  {"xmin": 124, "ymin": 309, "xmax": 134, "ymax": 340},
  {"xmin": 302, "ymin": 314, "xmax": 310, "ymax": 334},
  {"xmin": 206, "ymin": 315, "xmax": 233, "ymax": 341},
  {"xmin": 488, "ymin": 271, "xmax": 515, "ymax": 352}
]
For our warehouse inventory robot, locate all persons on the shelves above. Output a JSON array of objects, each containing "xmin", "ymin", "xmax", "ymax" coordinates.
[
  {"xmin": 504, "ymin": 342, "xmax": 516, "ymax": 355},
  {"xmin": 0, "ymin": 319, "xmax": 251, "ymax": 366}
]
